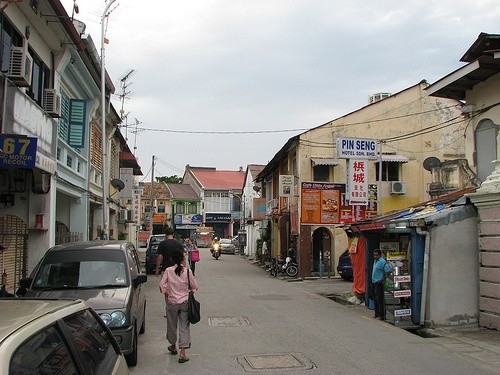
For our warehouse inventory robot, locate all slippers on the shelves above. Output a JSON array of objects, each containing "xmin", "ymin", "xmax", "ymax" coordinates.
[
  {"xmin": 179, "ymin": 355, "xmax": 189, "ymax": 363},
  {"xmin": 168, "ymin": 345, "xmax": 177, "ymax": 355}
]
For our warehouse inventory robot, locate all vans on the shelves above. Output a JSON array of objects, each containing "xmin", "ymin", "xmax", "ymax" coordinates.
[{"xmin": 16, "ymin": 240, "xmax": 147, "ymax": 366}]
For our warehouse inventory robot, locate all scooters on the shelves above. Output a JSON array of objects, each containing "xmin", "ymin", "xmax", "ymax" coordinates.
[{"xmin": 270, "ymin": 250, "xmax": 299, "ymax": 278}]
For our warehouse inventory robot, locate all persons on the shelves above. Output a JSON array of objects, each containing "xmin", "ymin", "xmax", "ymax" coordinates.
[
  {"xmin": 324, "ymin": 250, "xmax": 330, "ymax": 272},
  {"xmin": 183, "ymin": 238, "xmax": 197, "ymax": 277},
  {"xmin": 372, "ymin": 250, "xmax": 392, "ymax": 320},
  {"xmin": 156, "ymin": 227, "xmax": 185, "ymax": 318},
  {"xmin": 210, "ymin": 237, "xmax": 222, "ymax": 254},
  {"xmin": 159, "ymin": 249, "xmax": 198, "ymax": 363},
  {"xmin": 102, "ymin": 224, "xmax": 109, "ymax": 240},
  {"xmin": 262, "ymin": 238, "xmax": 273, "ymax": 266}
]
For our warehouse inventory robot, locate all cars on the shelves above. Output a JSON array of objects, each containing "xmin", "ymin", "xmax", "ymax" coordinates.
[
  {"xmin": 219, "ymin": 239, "xmax": 235, "ymax": 254},
  {"xmin": 145, "ymin": 234, "xmax": 169, "ymax": 275},
  {"xmin": 337, "ymin": 249, "xmax": 353, "ymax": 282},
  {"xmin": 0, "ymin": 296, "xmax": 131, "ymax": 375}
]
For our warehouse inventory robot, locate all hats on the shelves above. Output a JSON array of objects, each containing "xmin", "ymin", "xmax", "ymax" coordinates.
[{"xmin": 214, "ymin": 237, "xmax": 217, "ymax": 240}]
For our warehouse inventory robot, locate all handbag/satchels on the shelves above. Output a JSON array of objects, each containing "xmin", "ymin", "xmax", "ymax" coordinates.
[
  {"xmin": 188, "ymin": 249, "xmax": 200, "ymax": 261},
  {"xmin": 188, "ymin": 291, "xmax": 201, "ymax": 324}
]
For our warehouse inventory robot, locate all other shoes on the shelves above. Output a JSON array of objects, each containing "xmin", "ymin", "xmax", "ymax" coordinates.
[
  {"xmin": 379, "ymin": 317, "xmax": 387, "ymax": 321},
  {"xmin": 372, "ymin": 315, "xmax": 377, "ymax": 318}
]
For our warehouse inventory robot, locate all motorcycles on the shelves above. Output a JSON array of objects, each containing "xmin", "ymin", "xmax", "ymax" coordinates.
[{"xmin": 211, "ymin": 243, "xmax": 222, "ymax": 260}]
